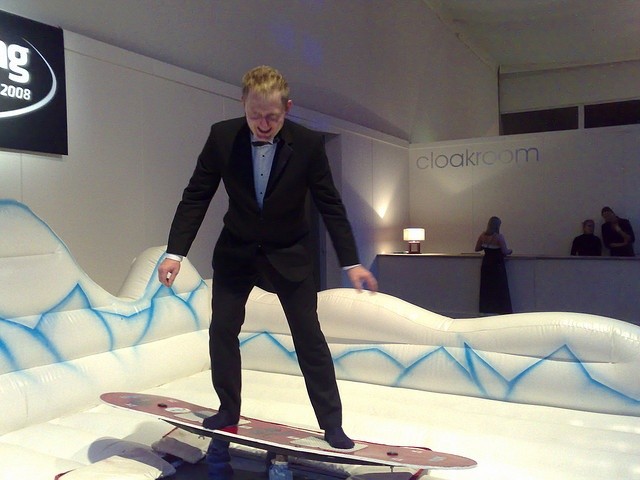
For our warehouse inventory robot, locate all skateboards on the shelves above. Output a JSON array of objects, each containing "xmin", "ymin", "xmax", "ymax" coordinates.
[{"xmin": 99, "ymin": 393, "xmax": 477, "ymax": 478}]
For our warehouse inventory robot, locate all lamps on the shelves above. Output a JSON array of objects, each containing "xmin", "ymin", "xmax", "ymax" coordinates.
[{"xmin": 400, "ymin": 226, "xmax": 427, "ymax": 253}]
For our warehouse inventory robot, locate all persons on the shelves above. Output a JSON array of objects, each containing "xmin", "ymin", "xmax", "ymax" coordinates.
[
  {"xmin": 158, "ymin": 64, "xmax": 378, "ymax": 449},
  {"xmin": 601, "ymin": 207, "xmax": 635, "ymax": 257},
  {"xmin": 570, "ymin": 219, "xmax": 602, "ymax": 256},
  {"xmin": 474, "ymin": 216, "xmax": 513, "ymax": 314}
]
